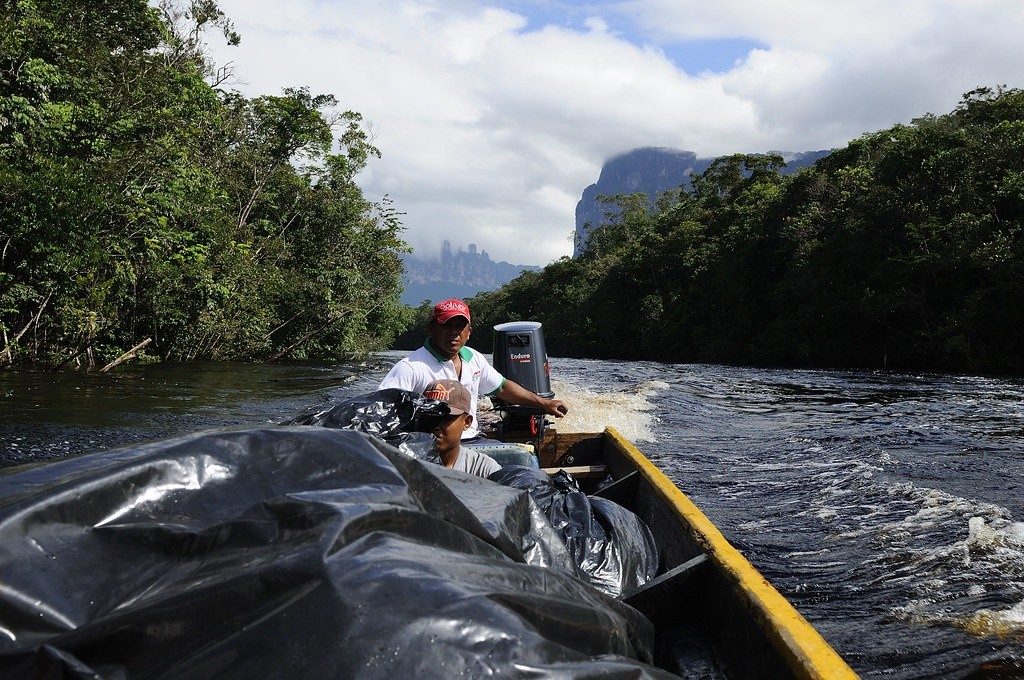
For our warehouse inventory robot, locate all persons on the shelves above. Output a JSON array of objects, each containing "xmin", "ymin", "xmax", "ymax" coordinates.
[
  {"xmin": 378, "ymin": 300, "xmax": 568, "ymax": 443},
  {"xmin": 422, "ymin": 380, "xmax": 502, "ymax": 479}
]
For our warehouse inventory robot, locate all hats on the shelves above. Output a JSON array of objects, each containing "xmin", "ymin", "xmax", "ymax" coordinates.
[
  {"xmin": 422, "ymin": 380, "xmax": 471, "ymax": 415},
  {"xmin": 431, "ymin": 298, "xmax": 471, "ymax": 325}
]
